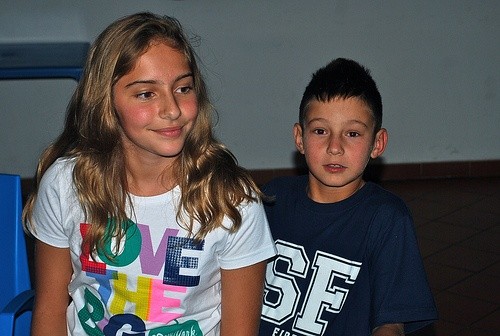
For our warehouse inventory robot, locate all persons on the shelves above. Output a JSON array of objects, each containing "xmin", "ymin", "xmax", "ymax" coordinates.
[
  {"xmin": 21, "ymin": 11, "xmax": 279, "ymax": 336},
  {"xmin": 246, "ymin": 58, "xmax": 439, "ymax": 336}
]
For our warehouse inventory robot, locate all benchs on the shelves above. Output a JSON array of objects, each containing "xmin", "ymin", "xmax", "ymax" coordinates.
[{"xmin": 248, "ymin": 159, "xmax": 499, "ymax": 336}]
